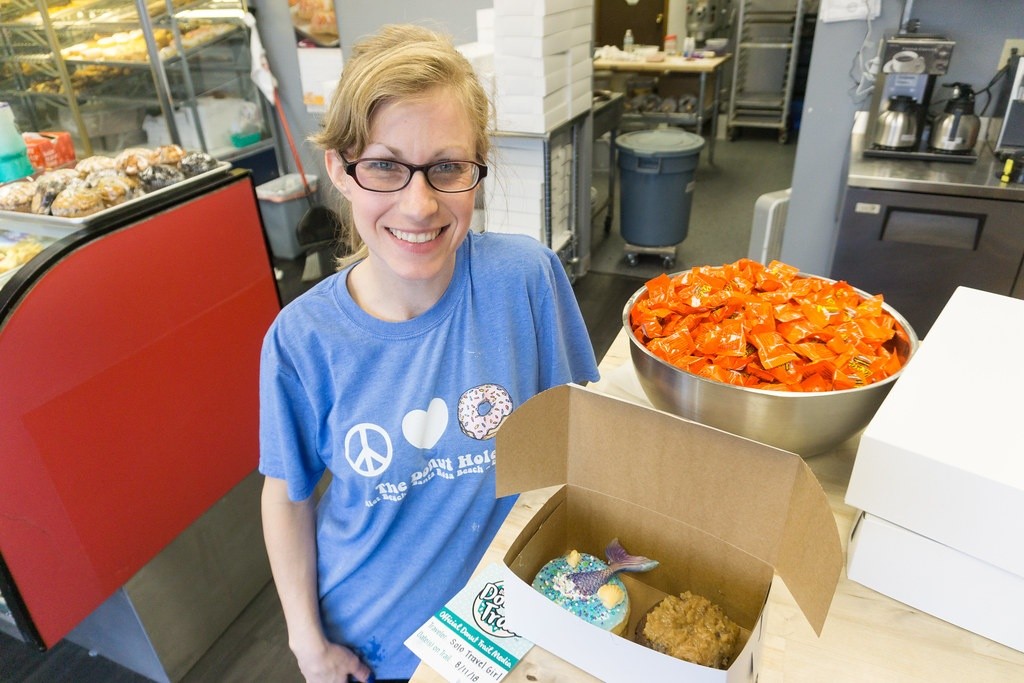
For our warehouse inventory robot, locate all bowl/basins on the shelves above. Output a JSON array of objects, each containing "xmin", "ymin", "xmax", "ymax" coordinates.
[{"xmin": 621, "ymin": 267, "xmax": 919, "ymax": 459}]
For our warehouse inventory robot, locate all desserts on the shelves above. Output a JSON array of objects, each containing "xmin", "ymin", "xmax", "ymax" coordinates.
[{"xmin": 529, "ymin": 549, "xmax": 742, "ymax": 671}]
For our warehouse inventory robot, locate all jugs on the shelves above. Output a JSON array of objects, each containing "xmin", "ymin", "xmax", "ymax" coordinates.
[
  {"xmin": 928, "ymin": 96, "xmax": 980, "ymax": 151},
  {"xmin": 873, "ymin": 94, "xmax": 925, "ymax": 151}
]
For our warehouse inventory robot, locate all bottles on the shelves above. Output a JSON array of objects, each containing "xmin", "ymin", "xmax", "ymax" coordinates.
[
  {"xmin": 0, "ymin": 101, "xmax": 34, "ymax": 184},
  {"xmin": 664, "ymin": 35, "xmax": 677, "ymax": 55},
  {"xmin": 623, "ymin": 29, "xmax": 633, "ymax": 59}
]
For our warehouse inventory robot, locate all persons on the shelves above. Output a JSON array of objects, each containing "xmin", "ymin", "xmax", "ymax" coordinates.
[{"xmin": 258, "ymin": 23, "xmax": 599, "ymax": 683}]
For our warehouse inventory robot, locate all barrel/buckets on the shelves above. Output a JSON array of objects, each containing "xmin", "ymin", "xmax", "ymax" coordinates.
[{"xmin": 615, "ymin": 130, "xmax": 706, "ymax": 247}]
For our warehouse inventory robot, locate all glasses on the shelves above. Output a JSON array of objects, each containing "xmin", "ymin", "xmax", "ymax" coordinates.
[{"xmin": 338, "ymin": 150, "xmax": 488, "ymax": 193}]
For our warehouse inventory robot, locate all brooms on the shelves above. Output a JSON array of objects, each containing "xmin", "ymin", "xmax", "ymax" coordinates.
[{"xmin": 272, "ymin": 86, "xmax": 352, "ymax": 281}]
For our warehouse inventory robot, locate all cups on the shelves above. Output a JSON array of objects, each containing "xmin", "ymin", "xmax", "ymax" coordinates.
[{"xmin": 893, "ymin": 50, "xmax": 923, "ymax": 71}]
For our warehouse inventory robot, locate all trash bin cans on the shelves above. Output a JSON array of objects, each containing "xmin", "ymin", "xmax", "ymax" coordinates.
[{"xmin": 255, "ymin": 174, "xmax": 319, "ymax": 260}]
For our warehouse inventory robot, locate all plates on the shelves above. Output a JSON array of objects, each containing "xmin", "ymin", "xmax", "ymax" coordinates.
[{"xmin": 883, "ymin": 58, "xmax": 927, "ymax": 73}]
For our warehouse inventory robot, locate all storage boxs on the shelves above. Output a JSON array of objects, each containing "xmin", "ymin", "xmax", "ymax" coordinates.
[
  {"xmin": 842, "ymin": 286, "xmax": 1024, "ymax": 578},
  {"xmin": 848, "ymin": 511, "xmax": 1023, "ymax": 651},
  {"xmin": 492, "ymin": 380, "xmax": 843, "ymax": 683}
]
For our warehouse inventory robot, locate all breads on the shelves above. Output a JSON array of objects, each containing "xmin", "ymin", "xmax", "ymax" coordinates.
[
  {"xmin": 0, "ymin": 145, "xmax": 218, "ymax": 274},
  {"xmin": 0, "ymin": 0, "xmax": 339, "ymax": 96}
]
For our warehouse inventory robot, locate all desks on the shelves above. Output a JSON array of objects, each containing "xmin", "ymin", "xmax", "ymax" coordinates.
[
  {"xmin": 591, "ymin": 48, "xmax": 734, "ymax": 165},
  {"xmin": 400, "ymin": 324, "xmax": 1023, "ymax": 683}
]
